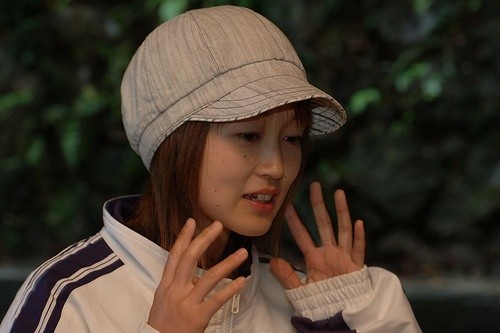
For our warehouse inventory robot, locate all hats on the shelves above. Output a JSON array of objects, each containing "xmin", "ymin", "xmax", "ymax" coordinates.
[{"xmin": 121, "ymin": 5, "xmax": 347, "ymax": 174}]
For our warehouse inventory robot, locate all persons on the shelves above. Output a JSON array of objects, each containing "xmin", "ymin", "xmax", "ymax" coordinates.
[{"xmin": 0, "ymin": 5, "xmax": 422, "ymax": 332}]
